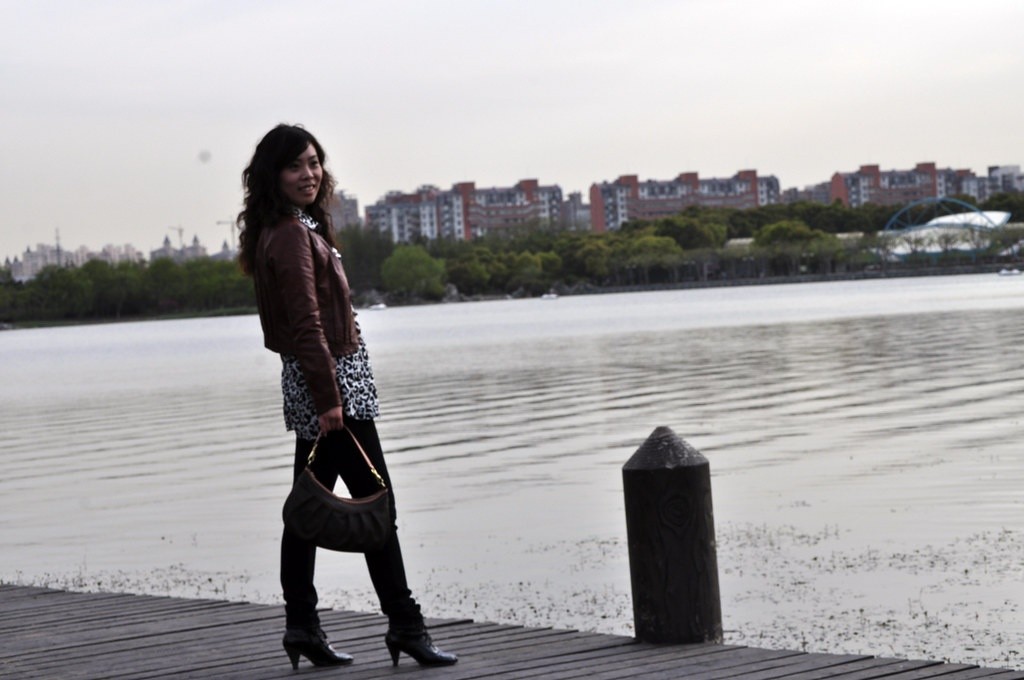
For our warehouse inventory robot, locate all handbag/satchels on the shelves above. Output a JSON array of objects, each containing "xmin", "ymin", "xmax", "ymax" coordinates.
[{"xmin": 278, "ymin": 421, "xmax": 395, "ymax": 555}]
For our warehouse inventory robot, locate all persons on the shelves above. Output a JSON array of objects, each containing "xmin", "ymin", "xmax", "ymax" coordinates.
[{"xmin": 237, "ymin": 125, "xmax": 458, "ymax": 670}]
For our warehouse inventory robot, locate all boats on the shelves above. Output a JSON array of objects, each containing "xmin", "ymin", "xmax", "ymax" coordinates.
[
  {"xmin": 371, "ymin": 302, "xmax": 388, "ymax": 311},
  {"xmin": 540, "ymin": 293, "xmax": 559, "ymax": 301},
  {"xmin": 997, "ymin": 266, "xmax": 1022, "ymax": 278}
]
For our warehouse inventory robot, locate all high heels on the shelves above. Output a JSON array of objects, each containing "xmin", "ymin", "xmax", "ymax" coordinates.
[
  {"xmin": 385, "ymin": 623, "xmax": 458, "ymax": 667},
  {"xmin": 282, "ymin": 625, "xmax": 353, "ymax": 670}
]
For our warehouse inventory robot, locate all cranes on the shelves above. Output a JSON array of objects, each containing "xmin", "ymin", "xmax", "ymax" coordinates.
[
  {"xmin": 172, "ymin": 226, "xmax": 186, "ymax": 251},
  {"xmin": 217, "ymin": 219, "xmax": 242, "ymax": 250}
]
